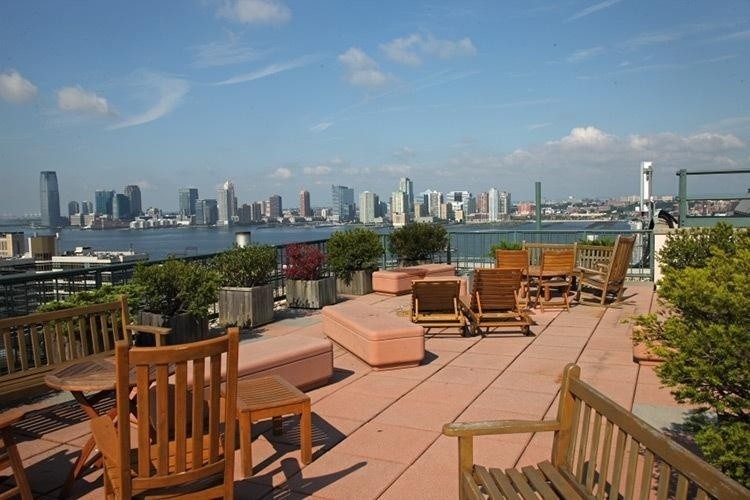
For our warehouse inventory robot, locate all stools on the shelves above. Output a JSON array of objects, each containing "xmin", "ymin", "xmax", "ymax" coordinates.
[{"xmin": 219, "ymin": 374, "xmax": 315, "ymax": 480}]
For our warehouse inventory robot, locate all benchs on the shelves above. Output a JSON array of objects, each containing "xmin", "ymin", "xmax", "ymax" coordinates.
[
  {"xmin": 1, "ymin": 293, "xmax": 173, "ymax": 405},
  {"xmin": 119, "ymin": 333, "xmax": 336, "ymax": 433},
  {"xmin": 319, "ymin": 299, "xmax": 426, "ymax": 373},
  {"xmin": 439, "ymin": 359, "xmax": 750, "ymax": 500}
]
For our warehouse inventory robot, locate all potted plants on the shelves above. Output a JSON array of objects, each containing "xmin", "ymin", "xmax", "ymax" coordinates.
[
  {"xmin": 325, "ymin": 227, "xmax": 375, "ymax": 295},
  {"xmin": 203, "ymin": 236, "xmax": 281, "ymax": 329},
  {"xmin": 132, "ymin": 252, "xmax": 217, "ymax": 349},
  {"xmin": 278, "ymin": 240, "xmax": 339, "ymax": 312}
]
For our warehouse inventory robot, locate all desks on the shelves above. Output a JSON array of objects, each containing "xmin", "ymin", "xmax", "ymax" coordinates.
[{"xmin": 43, "ymin": 352, "xmax": 182, "ymax": 499}]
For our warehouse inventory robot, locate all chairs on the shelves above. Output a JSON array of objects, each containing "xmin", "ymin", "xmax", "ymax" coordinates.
[
  {"xmin": 369, "ymin": 234, "xmax": 638, "ymax": 338},
  {"xmin": 1, "ymin": 406, "xmax": 35, "ymax": 500},
  {"xmin": 89, "ymin": 325, "xmax": 241, "ymax": 500}
]
